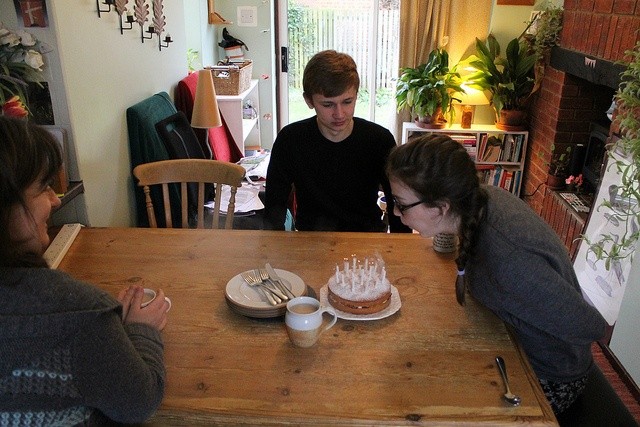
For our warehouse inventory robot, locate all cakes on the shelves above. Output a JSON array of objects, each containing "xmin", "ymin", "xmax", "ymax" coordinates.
[{"xmin": 328, "ymin": 254, "xmax": 392, "ymax": 315}]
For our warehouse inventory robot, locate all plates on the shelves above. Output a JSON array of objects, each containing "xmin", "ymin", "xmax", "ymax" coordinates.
[
  {"xmin": 320, "ymin": 277, "xmax": 401, "ymax": 320},
  {"xmin": 224, "ymin": 268, "xmax": 308, "ymax": 318}
]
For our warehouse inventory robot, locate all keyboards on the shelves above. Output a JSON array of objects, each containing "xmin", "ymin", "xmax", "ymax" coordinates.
[{"xmin": 559, "ymin": 192, "xmax": 591, "ymax": 213}]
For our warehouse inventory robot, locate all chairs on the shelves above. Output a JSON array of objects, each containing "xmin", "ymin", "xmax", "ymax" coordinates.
[{"xmin": 132, "ymin": 158, "xmax": 246, "ymax": 229}]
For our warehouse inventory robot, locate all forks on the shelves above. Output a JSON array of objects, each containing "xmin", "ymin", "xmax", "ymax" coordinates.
[{"xmin": 241, "ymin": 261, "xmax": 290, "ymax": 305}]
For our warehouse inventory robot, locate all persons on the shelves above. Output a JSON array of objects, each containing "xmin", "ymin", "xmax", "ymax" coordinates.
[
  {"xmin": 263, "ymin": 50, "xmax": 413, "ymax": 233},
  {"xmin": 0, "ymin": 115, "xmax": 170, "ymax": 426},
  {"xmin": 387, "ymin": 134, "xmax": 607, "ymax": 416}
]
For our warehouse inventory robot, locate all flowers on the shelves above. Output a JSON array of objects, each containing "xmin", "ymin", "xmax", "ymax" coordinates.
[{"xmin": 1, "ymin": 27, "xmax": 49, "ymax": 118}]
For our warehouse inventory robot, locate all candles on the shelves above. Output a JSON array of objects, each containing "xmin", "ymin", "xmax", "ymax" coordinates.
[
  {"xmin": 166, "ymin": 32, "xmax": 170, "ymax": 40},
  {"xmin": 148, "ymin": 23, "xmax": 155, "ymax": 31},
  {"xmin": 125, "ymin": 8, "xmax": 135, "ymax": 22}
]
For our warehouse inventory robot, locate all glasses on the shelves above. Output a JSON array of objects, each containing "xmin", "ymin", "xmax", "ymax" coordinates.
[{"xmin": 390, "ymin": 194, "xmax": 428, "ymax": 212}]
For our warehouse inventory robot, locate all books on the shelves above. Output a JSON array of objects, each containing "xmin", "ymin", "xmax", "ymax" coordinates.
[
  {"xmin": 447, "ymin": 133, "xmax": 524, "ymax": 162},
  {"xmin": 475, "ymin": 164, "xmax": 521, "ymax": 197}
]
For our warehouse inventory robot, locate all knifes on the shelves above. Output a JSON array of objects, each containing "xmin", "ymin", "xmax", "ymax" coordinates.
[{"xmin": 263, "ymin": 260, "xmax": 298, "ymax": 297}]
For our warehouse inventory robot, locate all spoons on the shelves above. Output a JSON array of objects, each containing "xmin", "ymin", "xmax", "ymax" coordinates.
[{"xmin": 495, "ymin": 356, "xmax": 522, "ymax": 406}]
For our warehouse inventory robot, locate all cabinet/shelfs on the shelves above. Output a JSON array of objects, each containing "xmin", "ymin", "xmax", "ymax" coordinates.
[
  {"xmin": 402, "ymin": 121, "xmax": 529, "ymax": 199},
  {"xmin": 216, "ymin": 79, "xmax": 262, "ymax": 156}
]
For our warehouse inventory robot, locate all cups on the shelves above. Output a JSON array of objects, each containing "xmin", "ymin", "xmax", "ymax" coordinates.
[
  {"xmin": 129, "ymin": 287, "xmax": 172, "ymax": 332},
  {"xmin": 432, "ymin": 232, "xmax": 456, "ymax": 253},
  {"xmin": 284, "ymin": 295, "xmax": 336, "ymax": 347}
]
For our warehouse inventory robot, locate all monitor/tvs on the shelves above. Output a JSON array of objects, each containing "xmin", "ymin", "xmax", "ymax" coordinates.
[{"xmin": 581, "ymin": 130, "xmax": 608, "ymax": 199}]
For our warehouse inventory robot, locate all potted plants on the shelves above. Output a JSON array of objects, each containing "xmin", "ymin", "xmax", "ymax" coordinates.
[
  {"xmin": 395, "ymin": 47, "xmax": 466, "ymax": 128},
  {"xmin": 467, "ymin": 30, "xmax": 541, "ymax": 130}
]
